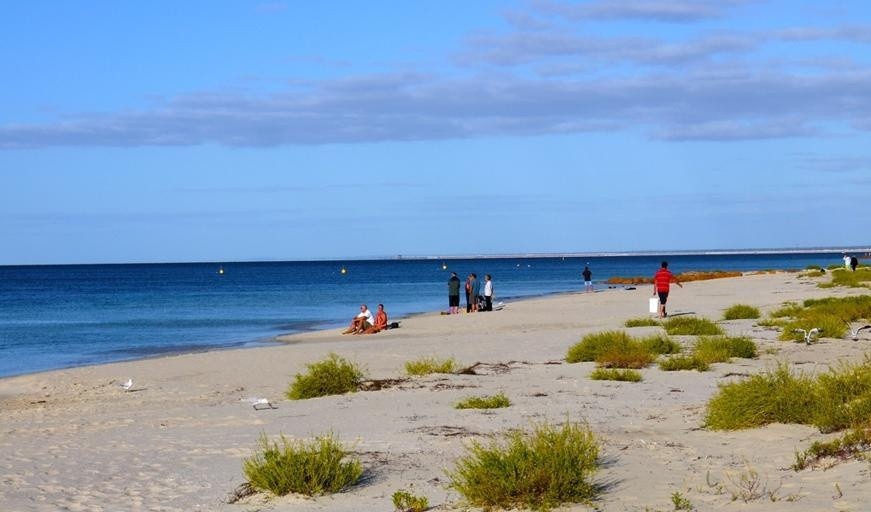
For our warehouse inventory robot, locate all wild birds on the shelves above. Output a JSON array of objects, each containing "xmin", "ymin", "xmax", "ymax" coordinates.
[
  {"xmin": 790, "ymin": 328, "xmax": 824, "ymax": 346},
  {"xmin": 841, "ymin": 318, "xmax": 871, "ymax": 340},
  {"xmin": 238, "ymin": 397, "xmax": 273, "ymax": 411},
  {"xmin": 119, "ymin": 378, "xmax": 134, "ymax": 393}
]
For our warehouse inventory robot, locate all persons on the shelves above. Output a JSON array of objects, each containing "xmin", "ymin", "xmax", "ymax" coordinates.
[
  {"xmin": 341, "ymin": 304, "xmax": 376, "ymax": 335},
  {"xmin": 363, "ymin": 303, "xmax": 388, "ymax": 334},
  {"xmin": 465, "ymin": 274, "xmax": 473, "ymax": 313},
  {"xmin": 653, "ymin": 260, "xmax": 684, "ymax": 319},
  {"xmin": 843, "ymin": 254, "xmax": 854, "ymax": 272},
  {"xmin": 447, "ymin": 271, "xmax": 460, "ymax": 313},
  {"xmin": 469, "ymin": 273, "xmax": 480, "ymax": 313},
  {"xmin": 850, "ymin": 255, "xmax": 859, "ymax": 272},
  {"xmin": 483, "ymin": 273, "xmax": 494, "ymax": 312},
  {"xmin": 583, "ymin": 266, "xmax": 594, "ymax": 294}
]
